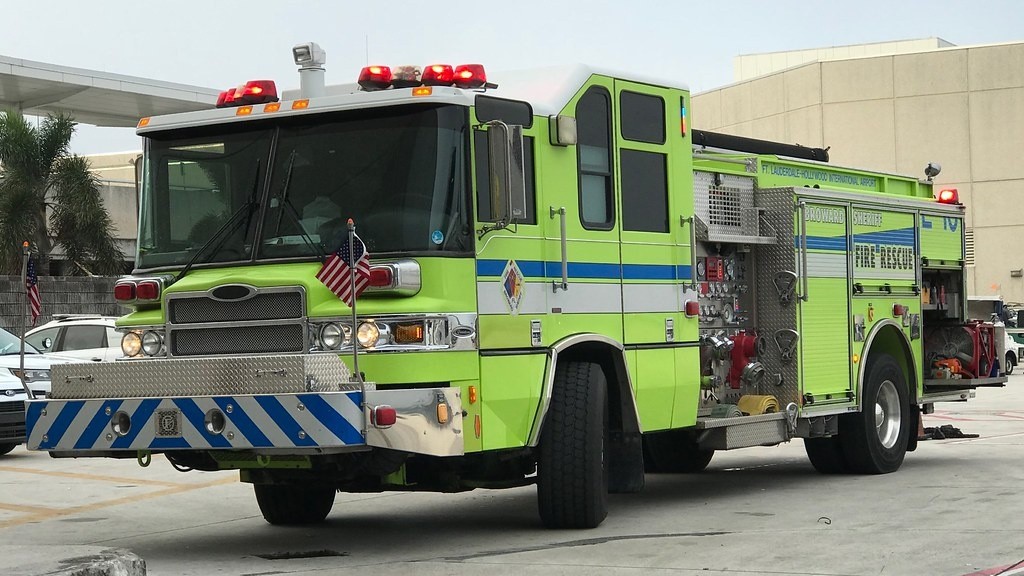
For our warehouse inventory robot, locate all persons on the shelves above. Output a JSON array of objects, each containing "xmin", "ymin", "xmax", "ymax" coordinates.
[{"xmin": 236, "ymin": 171, "xmax": 282, "ymax": 225}]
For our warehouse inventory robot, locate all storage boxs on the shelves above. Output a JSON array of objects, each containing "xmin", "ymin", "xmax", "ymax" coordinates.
[{"xmin": 921, "ymin": 287, "xmax": 931, "ymax": 303}]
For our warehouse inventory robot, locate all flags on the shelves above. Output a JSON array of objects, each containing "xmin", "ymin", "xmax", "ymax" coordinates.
[
  {"xmin": 315, "ymin": 232, "xmax": 371, "ymax": 307},
  {"xmin": 22, "ymin": 252, "xmax": 42, "ymax": 328}
]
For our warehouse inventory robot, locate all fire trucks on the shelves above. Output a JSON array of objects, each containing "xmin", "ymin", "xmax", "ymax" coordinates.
[{"xmin": 20, "ymin": 42, "xmax": 1006, "ymax": 530}]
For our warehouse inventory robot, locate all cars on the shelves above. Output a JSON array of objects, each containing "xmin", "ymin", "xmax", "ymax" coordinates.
[
  {"xmin": 1005, "ymin": 331, "xmax": 1018, "ymax": 376},
  {"xmin": 0, "ymin": 313, "xmax": 124, "ymax": 454}
]
